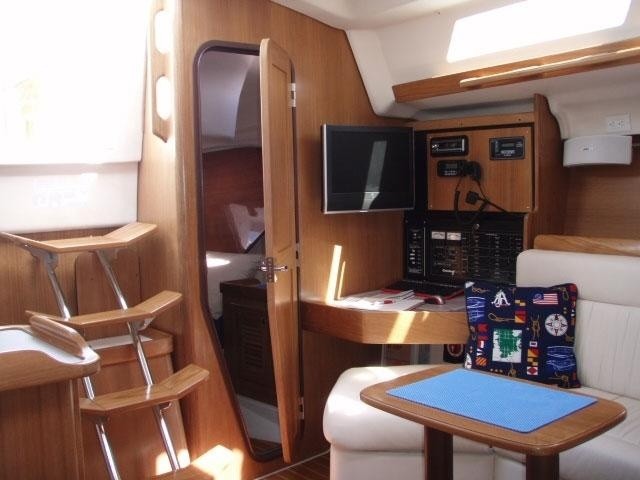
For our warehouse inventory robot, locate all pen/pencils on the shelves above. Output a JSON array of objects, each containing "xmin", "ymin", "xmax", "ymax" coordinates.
[{"xmin": 372, "ymin": 300, "xmax": 398, "ymax": 305}]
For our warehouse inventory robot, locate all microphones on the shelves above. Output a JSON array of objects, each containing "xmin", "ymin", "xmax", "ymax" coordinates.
[{"xmin": 464, "ymin": 191, "xmax": 508, "ymax": 212}]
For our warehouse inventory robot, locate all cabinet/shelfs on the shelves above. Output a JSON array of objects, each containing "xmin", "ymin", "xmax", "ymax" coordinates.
[{"xmin": 414, "ymin": 112, "xmax": 532, "ymax": 212}]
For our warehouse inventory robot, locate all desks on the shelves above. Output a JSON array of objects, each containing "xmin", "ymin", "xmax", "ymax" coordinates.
[
  {"xmin": 298, "ymin": 291, "xmax": 471, "ymax": 345},
  {"xmin": 360, "ymin": 364, "xmax": 626, "ymax": 480}
]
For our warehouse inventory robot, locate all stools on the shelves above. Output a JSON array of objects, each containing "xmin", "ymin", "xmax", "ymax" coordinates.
[{"xmin": 322, "ymin": 366, "xmax": 492, "ymax": 480}]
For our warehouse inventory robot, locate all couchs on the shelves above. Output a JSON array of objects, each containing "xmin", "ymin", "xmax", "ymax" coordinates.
[{"xmin": 487, "ymin": 251, "xmax": 640, "ymax": 480}]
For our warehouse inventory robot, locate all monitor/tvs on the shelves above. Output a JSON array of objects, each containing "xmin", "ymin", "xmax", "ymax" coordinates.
[{"xmin": 322, "ymin": 122, "xmax": 415, "ymax": 215}]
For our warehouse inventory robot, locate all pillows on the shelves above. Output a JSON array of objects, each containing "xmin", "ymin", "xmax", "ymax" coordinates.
[{"xmin": 463, "ymin": 280, "xmax": 581, "ymax": 389}]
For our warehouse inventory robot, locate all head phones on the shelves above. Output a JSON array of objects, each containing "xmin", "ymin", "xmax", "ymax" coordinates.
[{"xmin": 454, "ymin": 160, "xmax": 487, "ymax": 226}]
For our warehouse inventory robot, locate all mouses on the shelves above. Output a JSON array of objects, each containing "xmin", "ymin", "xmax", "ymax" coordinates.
[{"xmin": 425, "ymin": 295, "xmax": 445, "ymax": 304}]
